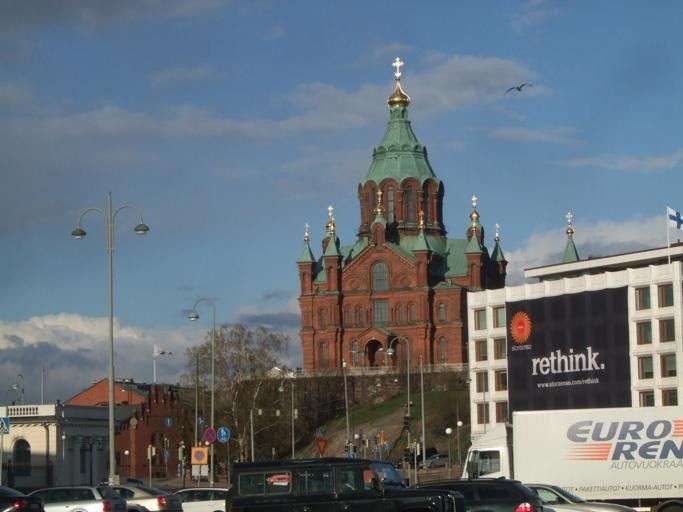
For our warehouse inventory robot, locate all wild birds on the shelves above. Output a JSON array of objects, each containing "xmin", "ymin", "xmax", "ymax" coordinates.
[{"xmin": 503, "ymin": 83, "xmax": 533, "ymax": 96}]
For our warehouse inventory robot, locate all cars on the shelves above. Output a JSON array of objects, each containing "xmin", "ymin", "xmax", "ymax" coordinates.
[
  {"xmin": 1, "ymin": 479, "xmax": 228, "ymax": 511},
  {"xmin": 409, "ymin": 479, "xmax": 542, "ymax": 512},
  {"xmin": 523, "ymin": 482, "xmax": 634, "ymax": 512}
]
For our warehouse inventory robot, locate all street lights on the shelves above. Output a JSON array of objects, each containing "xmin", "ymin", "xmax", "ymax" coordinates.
[
  {"xmin": 72, "ymin": 193, "xmax": 150, "ymax": 489},
  {"xmin": 278, "ymin": 375, "xmax": 296, "ymax": 459},
  {"xmin": 188, "ymin": 296, "xmax": 217, "ymax": 488},
  {"xmin": 11, "ymin": 372, "xmax": 25, "ymax": 405},
  {"xmin": 386, "ymin": 334, "xmax": 412, "ymax": 487},
  {"xmin": 445, "ymin": 420, "xmax": 463, "ymax": 468},
  {"xmin": 465, "ymin": 367, "xmax": 486, "ymax": 433}
]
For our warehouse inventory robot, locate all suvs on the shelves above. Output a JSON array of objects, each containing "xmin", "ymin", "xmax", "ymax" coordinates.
[{"xmin": 226, "ymin": 455, "xmax": 466, "ymax": 512}]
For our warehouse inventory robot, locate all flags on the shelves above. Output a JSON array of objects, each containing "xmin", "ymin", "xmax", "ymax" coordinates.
[{"xmin": 669, "ymin": 208, "xmax": 683, "ymax": 231}]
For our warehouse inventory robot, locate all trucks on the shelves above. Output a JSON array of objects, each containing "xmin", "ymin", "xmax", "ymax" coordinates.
[{"xmin": 460, "ymin": 405, "xmax": 683, "ymax": 511}]
